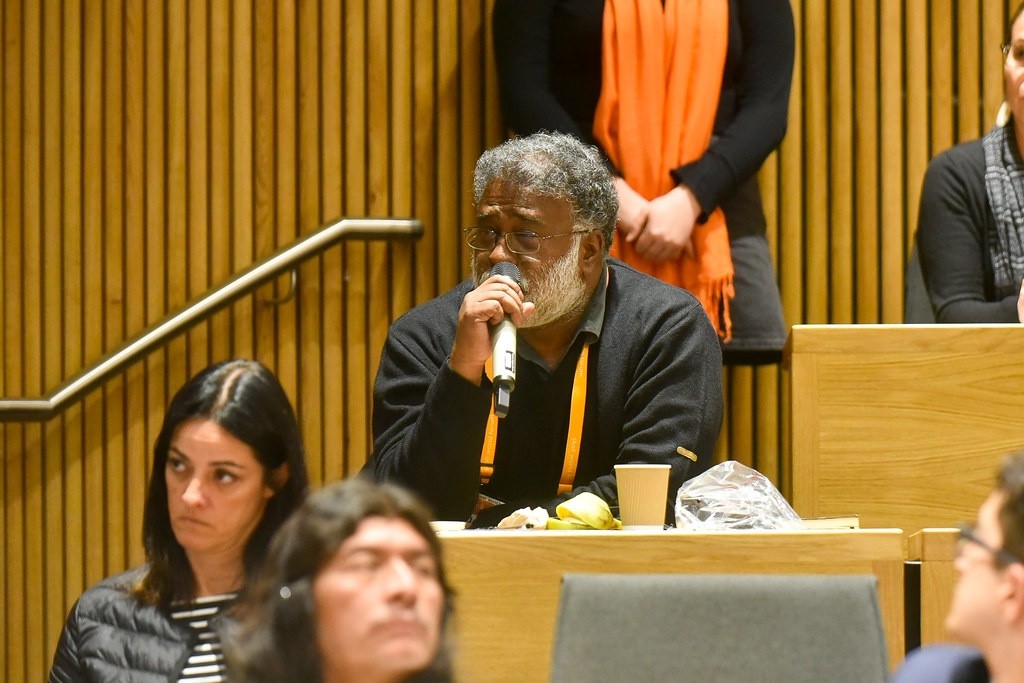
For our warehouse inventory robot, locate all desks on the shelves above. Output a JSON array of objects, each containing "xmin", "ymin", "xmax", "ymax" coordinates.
[{"xmin": 442, "ymin": 320, "xmax": 1024, "ymax": 682}]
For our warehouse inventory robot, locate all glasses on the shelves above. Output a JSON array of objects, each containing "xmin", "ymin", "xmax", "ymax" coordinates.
[
  {"xmin": 464, "ymin": 224, "xmax": 599, "ymax": 255},
  {"xmin": 1001, "ymin": 41, "xmax": 1024, "ymax": 67},
  {"xmin": 955, "ymin": 520, "xmax": 1017, "ymax": 569}
]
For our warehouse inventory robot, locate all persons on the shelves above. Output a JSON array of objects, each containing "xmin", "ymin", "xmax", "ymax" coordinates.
[
  {"xmin": 222, "ymin": 477, "xmax": 464, "ymax": 683},
  {"xmin": 490, "ymin": 1, "xmax": 789, "ymax": 365},
  {"xmin": 49, "ymin": 360, "xmax": 310, "ymax": 683},
  {"xmin": 888, "ymin": 452, "xmax": 1022, "ymax": 682},
  {"xmin": 918, "ymin": 1, "xmax": 1022, "ymax": 325},
  {"xmin": 355, "ymin": 129, "xmax": 723, "ymax": 531}
]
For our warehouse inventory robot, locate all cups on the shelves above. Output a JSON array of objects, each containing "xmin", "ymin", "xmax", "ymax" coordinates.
[{"xmin": 613, "ymin": 465, "xmax": 672, "ymax": 532}]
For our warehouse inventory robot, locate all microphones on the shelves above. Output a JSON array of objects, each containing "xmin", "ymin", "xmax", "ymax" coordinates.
[{"xmin": 490, "ymin": 261, "xmax": 522, "ymax": 418}]
